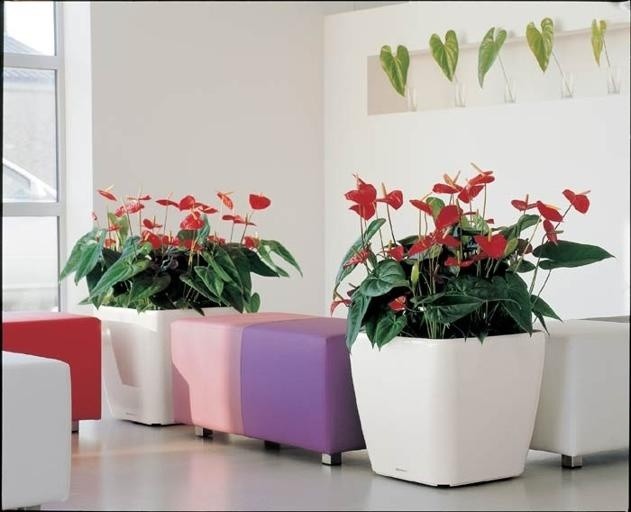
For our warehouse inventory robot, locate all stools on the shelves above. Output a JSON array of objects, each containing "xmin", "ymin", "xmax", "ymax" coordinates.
[
  {"xmin": 530, "ymin": 313, "xmax": 631, "ymax": 469},
  {"xmin": 1, "ymin": 311, "xmax": 103, "ymax": 512},
  {"xmin": 170, "ymin": 312, "xmax": 366, "ymax": 467}
]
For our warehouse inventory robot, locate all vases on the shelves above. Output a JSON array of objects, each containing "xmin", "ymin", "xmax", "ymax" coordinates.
[
  {"xmin": 349, "ymin": 329, "xmax": 545, "ymax": 488},
  {"xmin": 94, "ymin": 303, "xmax": 240, "ymax": 426}
]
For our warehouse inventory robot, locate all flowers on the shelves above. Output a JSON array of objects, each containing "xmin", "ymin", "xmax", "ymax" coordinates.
[
  {"xmin": 330, "ymin": 163, "xmax": 616, "ymax": 350},
  {"xmin": 56, "ymin": 188, "xmax": 303, "ymax": 314}
]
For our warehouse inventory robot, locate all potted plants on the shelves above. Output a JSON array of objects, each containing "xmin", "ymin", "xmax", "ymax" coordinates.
[{"xmin": 379, "ymin": 17, "xmax": 626, "ymax": 114}]
